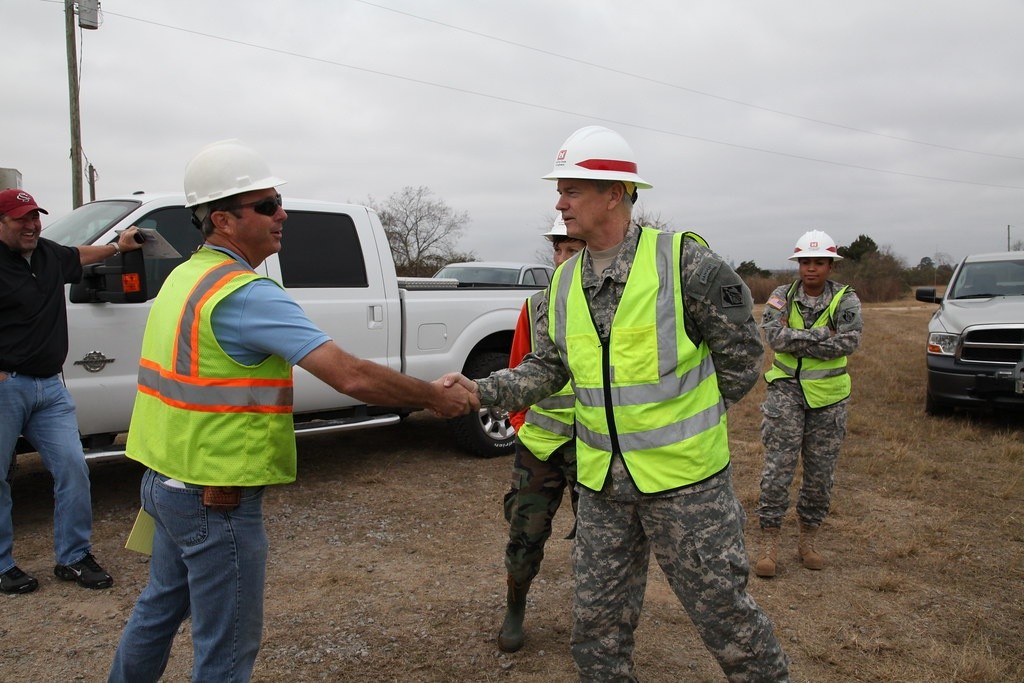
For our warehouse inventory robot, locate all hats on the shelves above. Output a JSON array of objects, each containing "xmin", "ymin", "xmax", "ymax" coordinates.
[{"xmin": 0, "ymin": 187, "xmax": 50, "ymax": 219}]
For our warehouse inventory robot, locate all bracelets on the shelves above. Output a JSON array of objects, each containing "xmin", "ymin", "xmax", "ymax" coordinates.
[{"xmin": 109, "ymin": 242, "xmax": 120, "ymax": 257}]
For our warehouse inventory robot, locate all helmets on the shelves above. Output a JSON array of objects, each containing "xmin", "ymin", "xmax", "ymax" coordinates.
[
  {"xmin": 540, "ymin": 211, "xmax": 568, "ymax": 242},
  {"xmin": 184, "ymin": 139, "xmax": 286, "ymax": 207},
  {"xmin": 788, "ymin": 230, "xmax": 844, "ymax": 261},
  {"xmin": 541, "ymin": 126, "xmax": 654, "ymax": 189}
]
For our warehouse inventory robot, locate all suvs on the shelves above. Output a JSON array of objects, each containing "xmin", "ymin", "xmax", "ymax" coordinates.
[{"xmin": 915, "ymin": 250, "xmax": 1024, "ymax": 414}]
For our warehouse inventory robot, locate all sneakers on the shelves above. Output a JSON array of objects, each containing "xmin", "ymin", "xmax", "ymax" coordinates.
[
  {"xmin": 0, "ymin": 567, "xmax": 38, "ymax": 594},
  {"xmin": 54, "ymin": 551, "xmax": 113, "ymax": 589}
]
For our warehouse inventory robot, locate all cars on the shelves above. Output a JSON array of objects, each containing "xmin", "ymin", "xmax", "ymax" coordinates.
[{"xmin": 431, "ymin": 261, "xmax": 555, "ymax": 286}]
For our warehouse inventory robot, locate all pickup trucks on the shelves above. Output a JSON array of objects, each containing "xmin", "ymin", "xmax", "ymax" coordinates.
[{"xmin": 15, "ymin": 191, "xmax": 548, "ymax": 457}]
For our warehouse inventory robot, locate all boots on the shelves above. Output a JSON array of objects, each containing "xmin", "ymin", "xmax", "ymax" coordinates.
[
  {"xmin": 755, "ymin": 526, "xmax": 781, "ymax": 576},
  {"xmin": 797, "ymin": 526, "xmax": 822, "ymax": 569},
  {"xmin": 498, "ymin": 576, "xmax": 532, "ymax": 653}
]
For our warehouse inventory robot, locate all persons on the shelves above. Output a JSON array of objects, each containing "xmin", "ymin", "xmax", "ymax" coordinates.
[
  {"xmin": 429, "ymin": 124, "xmax": 794, "ymax": 683},
  {"xmin": 0, "ymin": 186, "xmax": 146, "ymax": 594},
  {"xmin": 756, "ymin": 229, "xmax": 865, "ymax": 577},
  {"xmin": 499, "ymin": 214, "xmax": 586, "ymax": 654},
  {"xmin": 108, "ymin": 136, "xmax": 480, "ymax": 683}
]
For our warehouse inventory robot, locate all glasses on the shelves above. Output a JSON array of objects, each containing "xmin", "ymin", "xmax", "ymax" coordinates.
[{"xmin": 233, "ymin": 194, "xmax": 283, "ymax": 217}]
